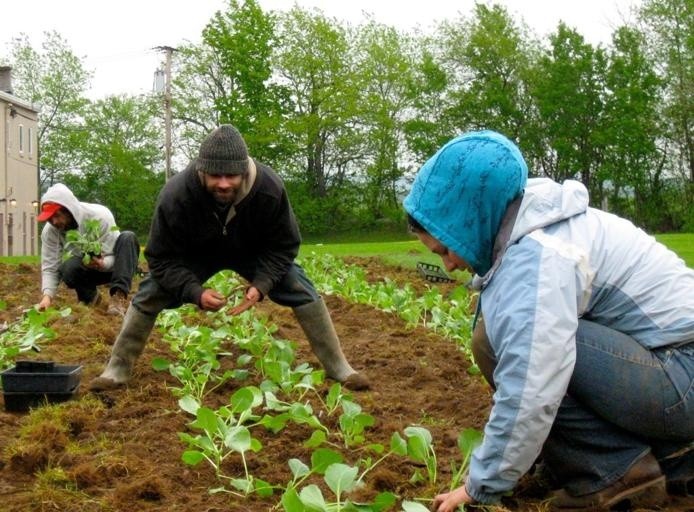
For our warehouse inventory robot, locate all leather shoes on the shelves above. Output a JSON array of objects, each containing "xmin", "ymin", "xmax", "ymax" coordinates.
[
  {"xmin": 550, "ymin": 452, "xmax": 666, "ymax": 511},
  {"xmin": 513, "ymin": 470, "xmax": 568, "ymax": 502}
]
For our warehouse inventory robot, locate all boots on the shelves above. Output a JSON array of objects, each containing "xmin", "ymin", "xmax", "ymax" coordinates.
[
  {"xmin": 87, "ymin": 301, "xmax": 155, "ymax": 389},
  {"xmin": 293, "ymin": 295, "xmax": 370, "ymax": 390}
]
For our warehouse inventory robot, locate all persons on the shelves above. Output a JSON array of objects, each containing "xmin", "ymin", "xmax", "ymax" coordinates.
[
  {"xmin": 86, "ymin": 124, "xmax": 370, "ymax": 392},
  {"xmin": 401, "ymin": 128, "xmax": 693, "ymax": 512},
  {"xmin": 33, "ymin": 182, "xmax": 140, "ymax": 312}
]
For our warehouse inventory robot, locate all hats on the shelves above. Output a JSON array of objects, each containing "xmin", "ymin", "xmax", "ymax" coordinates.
[
  {"xmin": 195, "ymin": 124, "xmax": 249, "ymax": 175},
  {"xmin": 37, "ymin": 202, "xmax": 62, "ymax": 222}
]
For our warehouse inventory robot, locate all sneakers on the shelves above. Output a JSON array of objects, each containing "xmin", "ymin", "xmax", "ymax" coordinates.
[
  {"xmin": 107, "ymin": 291, "xmax": 125, "ymax": 317},
  {"xmin": 78, "ymin": 289, "xmax": 102, "ymax": 307}
]
